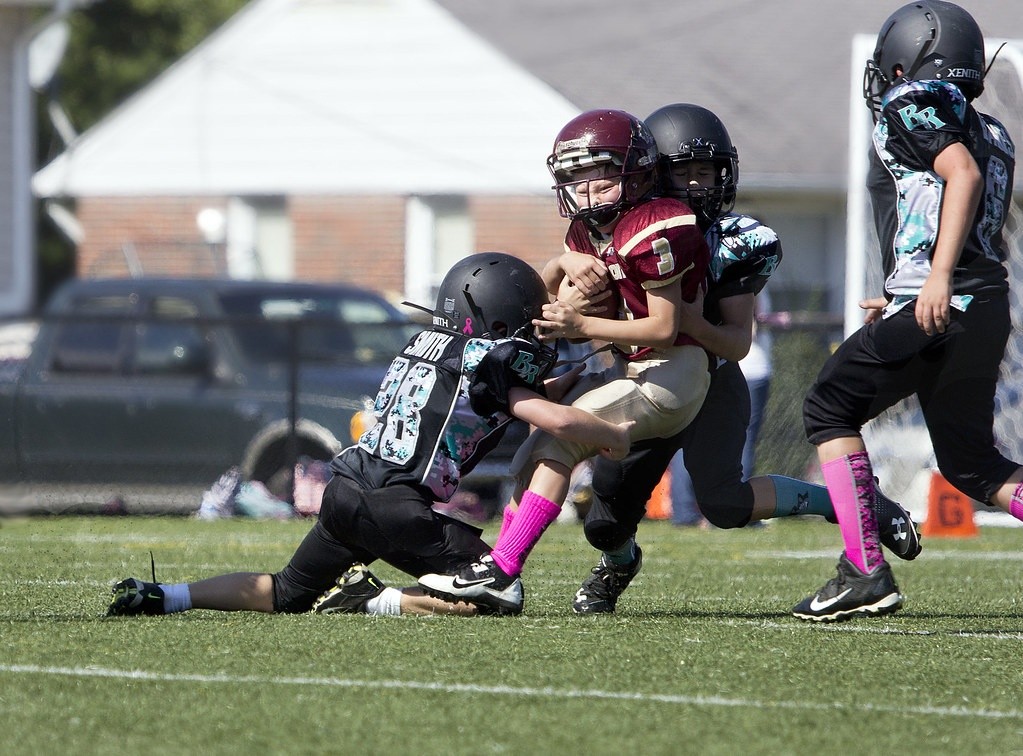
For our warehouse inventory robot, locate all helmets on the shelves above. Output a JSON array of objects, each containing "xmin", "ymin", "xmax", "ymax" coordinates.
[
  {"xmin": 548, "ymin": 108, "xmax": 660, "ymax": 228},
  {"xmin": 401, "ymin": 252, "xmax": 551, "ymax": 341},
  {"xmin": 645, "ymin": 103, "xmax": 738, "ymax": 235},
  {"xmin": 861, "ymin": 0, "xmax": 1007, "ymax": 124}
]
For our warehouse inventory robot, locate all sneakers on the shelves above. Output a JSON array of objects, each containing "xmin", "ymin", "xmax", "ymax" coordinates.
[
  {"xmin": 872, "ymin": 477, "xmax": 922, "ymax": 559},
  {"xmin": 572, "ymin": 545, "xmax": 642, "ymax": 618},
  {"xmin": 309, "ymin": 562, "xmax": 386, "ymax": 617},
  {"xmin": 793, "ymin": 550, "xmax": 903, "ymax": 622},
  {"xmin": 105, "ymin": 552, "xmax": 166, "ymax": 617},
  {"xmin": 417, "ymin": 550, "xmax": 525, "ymax": 616}
]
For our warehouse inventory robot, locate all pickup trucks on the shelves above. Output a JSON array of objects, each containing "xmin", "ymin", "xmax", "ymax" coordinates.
[{"xmin": 0, "ymin": 273, "xmax": 533, "ymax": 517}]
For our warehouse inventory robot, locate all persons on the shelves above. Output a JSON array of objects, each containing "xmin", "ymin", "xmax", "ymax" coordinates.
[
  {"xmin": 107, "ymin": 103, "xmax": 922, "ymax": 617},
  {"xmin": 792, "ymin": 0, "xmax": 1023, "ymax": 624}
]
